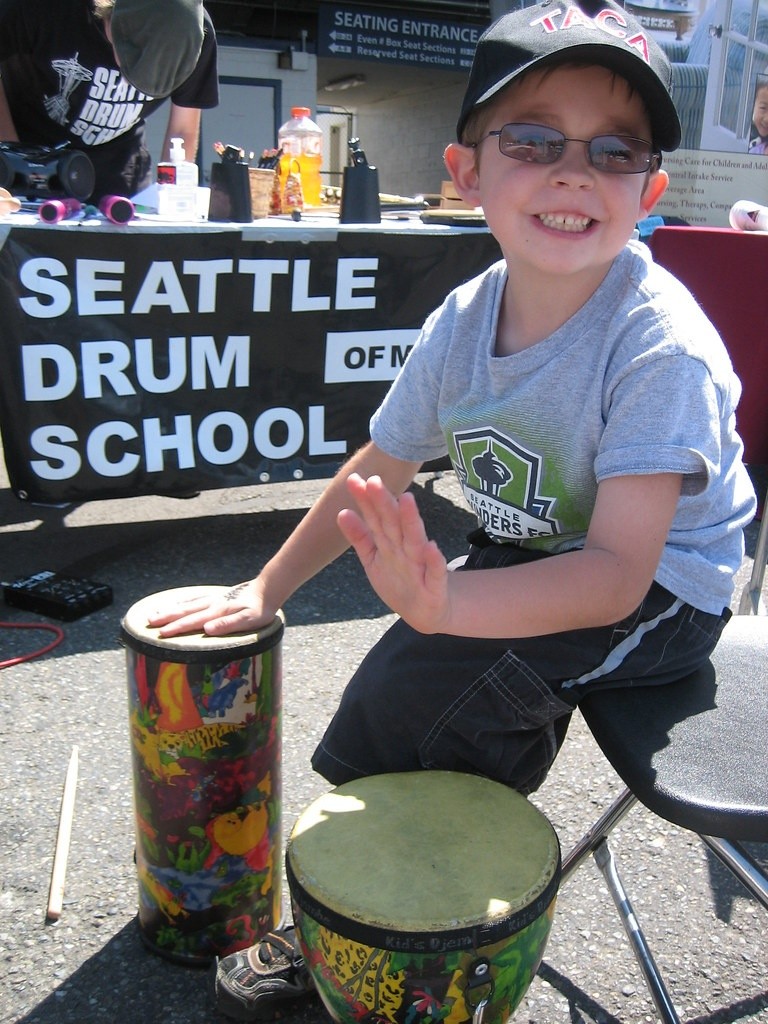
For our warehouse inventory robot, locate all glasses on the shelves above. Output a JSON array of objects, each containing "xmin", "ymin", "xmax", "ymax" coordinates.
[{"xmin": 469, "ymin": 121, "xmax": 661, "ymax": 173}]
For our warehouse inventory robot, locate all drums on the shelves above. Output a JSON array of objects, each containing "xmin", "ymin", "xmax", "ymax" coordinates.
[
  {"xmin": 287, "ymin": 771, "xmax": 562, "ymax": 1024},
  {"xmin": 120, "ymin": 585, "xmax": 285, "ymax": 968}
]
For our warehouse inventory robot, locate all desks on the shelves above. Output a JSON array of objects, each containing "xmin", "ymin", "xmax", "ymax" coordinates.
[
  {"xmin": 649, "ymin": 225, "xmax": 767, "ymax": 480},
  {"xmin": 0, "ymin": 200, "xmax": 509, "ymax": 523}
]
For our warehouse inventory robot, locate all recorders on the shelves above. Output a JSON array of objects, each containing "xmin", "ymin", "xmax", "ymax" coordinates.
[{"xmin": 0, "ymin": 142, "xmax": 96, "ymax": 201}]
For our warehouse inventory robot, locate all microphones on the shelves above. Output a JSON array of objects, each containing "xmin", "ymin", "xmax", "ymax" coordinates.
[
  {"xmin": 100, "ymin": 193, "xmax": 134, "ymax": 223},
  {"xmin": 39, "ymin": 198, "xmax": 82, "ymax": 224}
]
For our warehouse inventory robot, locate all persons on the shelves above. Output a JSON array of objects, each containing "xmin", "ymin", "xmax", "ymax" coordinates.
[
  {"xmin": 749, "ymin": 80, "xmax": 767, "ymax": 143},
  {"xmin": 152, "ymin": 1, "xmax": 759, "ymax": 1023},
  {"xmin": 1, "ymin": 1, "xmax": 222, "ymax": 202}
]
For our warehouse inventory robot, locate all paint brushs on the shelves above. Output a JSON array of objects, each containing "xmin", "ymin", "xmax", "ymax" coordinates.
[{"xmin": 212, "ymin": 141, "xmax": 285, "ymax": 170}]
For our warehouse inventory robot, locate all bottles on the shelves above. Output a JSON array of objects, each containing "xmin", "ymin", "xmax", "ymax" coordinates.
[
  {"xmin": 157, "ymin": 137, "xmax": 200, "ymax": 221},
  {"xmin": 275, "ymin": 105, "xmax": 326, "ymax": 211}
]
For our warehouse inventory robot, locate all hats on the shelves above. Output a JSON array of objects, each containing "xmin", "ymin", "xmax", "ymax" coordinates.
[
  {"xmin": 110, "ymin": 0, "xmax": 204, "ymax": 100},
  {"xmin": 457, "ymin": 0, "xmax": 682, "ymax": 152}
]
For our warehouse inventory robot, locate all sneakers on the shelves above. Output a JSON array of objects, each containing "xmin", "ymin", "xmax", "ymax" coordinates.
[{"xmin": 213, "ymin": 925, "xmax": 324, "ymax": 1022}]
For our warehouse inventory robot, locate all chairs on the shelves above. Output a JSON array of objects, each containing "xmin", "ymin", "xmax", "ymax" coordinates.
[{"xmin": 495, "ymin": 495, "xmax": 768, "ymax": 1022}]
